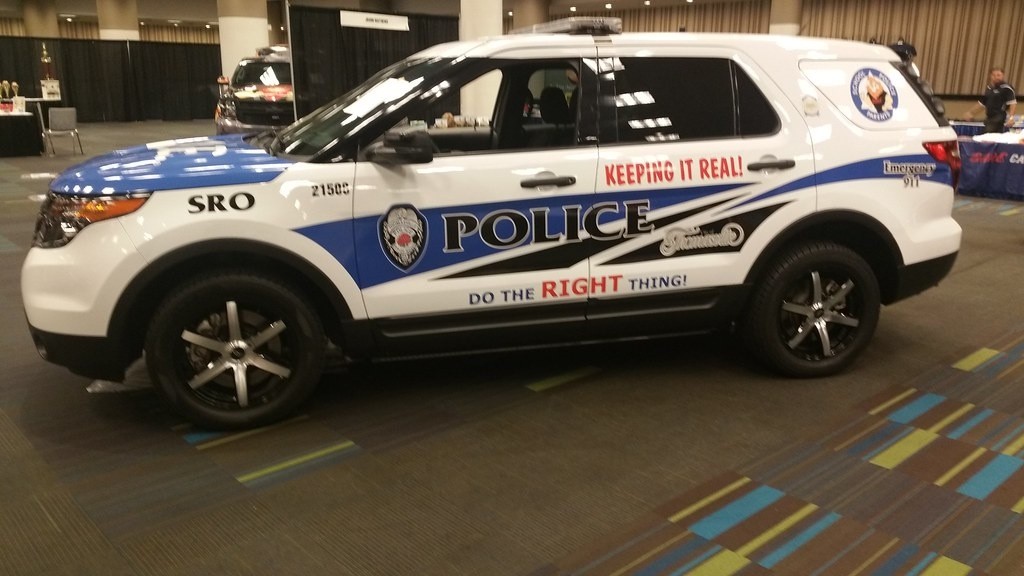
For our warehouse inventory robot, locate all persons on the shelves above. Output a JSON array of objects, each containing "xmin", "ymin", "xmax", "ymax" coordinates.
[{"xmin": 966, "ymin": 68, "xmax": 1017, "ymax": 134}]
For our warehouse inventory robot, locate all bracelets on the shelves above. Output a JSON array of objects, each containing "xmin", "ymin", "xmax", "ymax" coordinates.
[{"xmin": 1009, "ymin": 118, "xmax": 1014, "ymax": 121}]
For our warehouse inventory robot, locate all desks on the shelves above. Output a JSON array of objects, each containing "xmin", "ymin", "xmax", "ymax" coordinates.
[{"xmin": 950, "ymin": 119, "xmax": 1024, "ymax": 198}]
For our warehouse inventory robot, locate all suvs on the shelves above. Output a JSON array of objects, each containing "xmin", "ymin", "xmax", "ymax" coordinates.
[
  {"xmin": 214, "ymin": 55, "xmax": 296, "ymax": 136},
  {"xmin": 19, "ymin": 15, "xmax": 963, "ymax": 433}
]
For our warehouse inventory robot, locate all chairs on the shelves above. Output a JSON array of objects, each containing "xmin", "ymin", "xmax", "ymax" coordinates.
[{"xmin": 39, "ymin": 107, "xmax": 86, "ymax": 157}]
